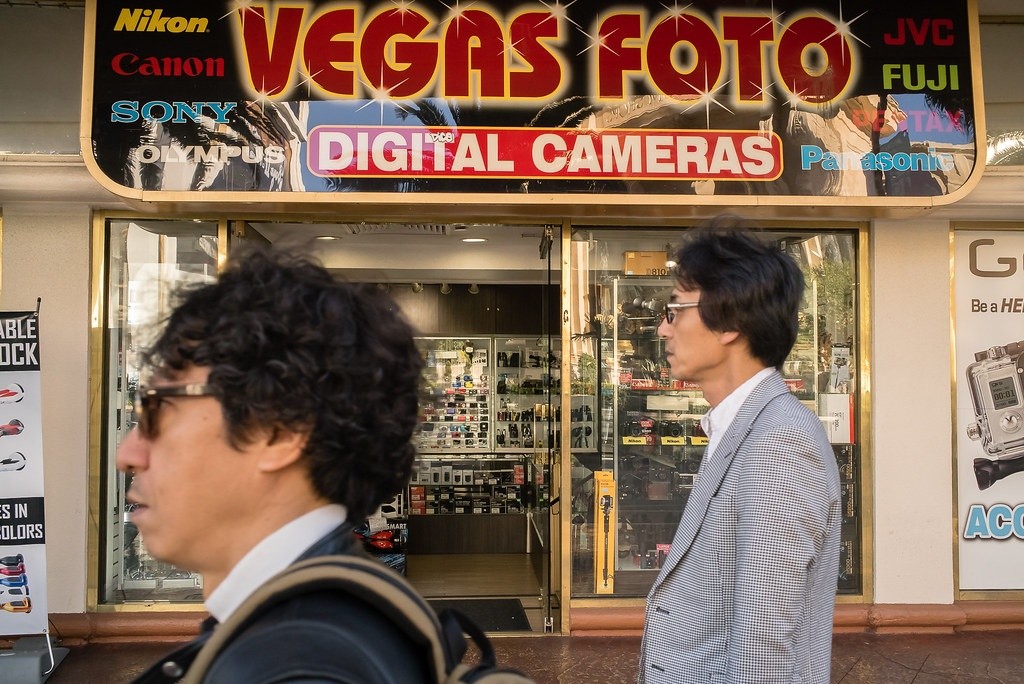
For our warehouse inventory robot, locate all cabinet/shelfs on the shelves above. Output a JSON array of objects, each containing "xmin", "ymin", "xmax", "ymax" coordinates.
[
  {"xmin": 495, "ymin": 336, "xmax": 600, "ymax": 451},
  {"xmin": 411, "ymin": 335, "xmax": 492, "ymax": 452},
  {"xmin": 599, "ymin": 275, "xmax": 822, "ymax": 573}
]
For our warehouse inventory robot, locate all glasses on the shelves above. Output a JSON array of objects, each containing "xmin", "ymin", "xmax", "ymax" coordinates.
[
  {"xmin": 134, "ymin": 385, "xmax": 218, "ymax": 441},
  {"xmin": 665, "ymin": 302, "xmax": 699, "ymax": 325}
]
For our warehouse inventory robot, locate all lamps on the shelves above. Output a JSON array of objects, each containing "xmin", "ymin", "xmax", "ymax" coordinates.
[
  {"xmin": 441, "ymin": 283, "xmax": 452, "ymax": 295},
  {"xmin": 412, "ymin": 282, "xmax": 423, "ymax": 293},
  {"xmin": 468, "ymin": 282, "xmax": 480, "ymax": 295}
]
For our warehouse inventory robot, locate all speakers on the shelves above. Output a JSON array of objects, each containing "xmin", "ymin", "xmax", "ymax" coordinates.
[{"xmin": 641, "ymin": 557, "xmax": 657, "ymax": 570}]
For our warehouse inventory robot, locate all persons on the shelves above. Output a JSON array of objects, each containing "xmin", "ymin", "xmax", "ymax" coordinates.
[
  {"xmin": 635, "ymin": 228, "xmax": 841, "ymax": 684},
  {"xmin": 114, "ymin": 245, "xmax": 437, "ymax": 684}
]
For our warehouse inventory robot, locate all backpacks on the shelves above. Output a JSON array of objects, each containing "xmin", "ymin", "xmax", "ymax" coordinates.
[{"xmin": 177, "ymin": 554, "xmax": 533, "ymax": 684}]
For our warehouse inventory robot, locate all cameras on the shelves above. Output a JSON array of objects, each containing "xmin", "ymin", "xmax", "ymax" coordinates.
[{"xmin": 619, "ymin": 415, "xmax": 708, "ymax": 502}]
